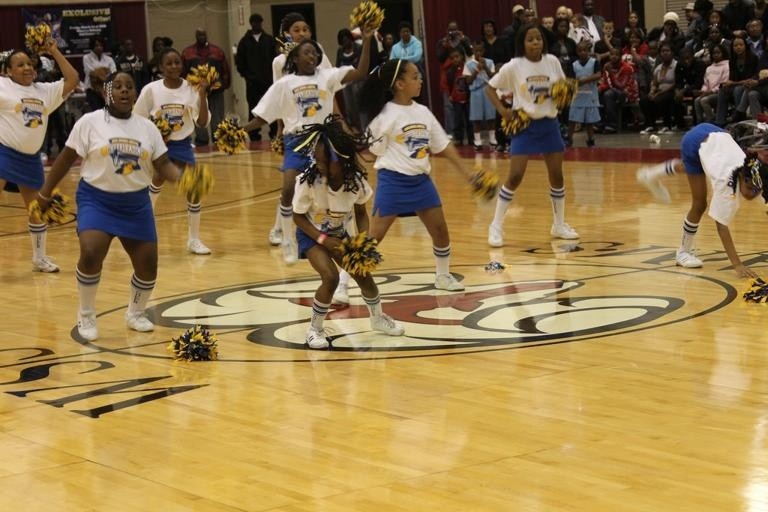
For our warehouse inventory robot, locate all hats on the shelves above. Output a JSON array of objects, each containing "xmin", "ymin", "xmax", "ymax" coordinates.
[
  {"xmin": 512, "ymin": 4, "xmax": 525, "ymax": 14},
  {"xmin": 663, "ymin": 12, "xmax": 680, "ymax": 25},
  {"xmin": 681, "ymin": 2, "xmax": 696, "ymax": 11}
]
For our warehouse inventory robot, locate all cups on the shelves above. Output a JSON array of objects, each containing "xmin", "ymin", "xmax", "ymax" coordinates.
[{"xmin": 648, "ymin": 134, "xmax": 660, "ymax": 144}]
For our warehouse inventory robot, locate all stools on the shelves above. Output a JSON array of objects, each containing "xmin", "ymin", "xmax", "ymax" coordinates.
[{"xmin": 608, "ymin": 90, "xmax": 699, "ymax": 132}]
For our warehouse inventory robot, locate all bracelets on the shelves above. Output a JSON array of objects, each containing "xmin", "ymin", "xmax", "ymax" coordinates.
[{"xmin": 38, "ymin": 192, "xmax": 51, "ymax": 202}]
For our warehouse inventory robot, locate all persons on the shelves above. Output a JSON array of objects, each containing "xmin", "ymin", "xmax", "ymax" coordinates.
[
  {"xmin": 290, "ymin": 113, "xmax": 403, "ymax": 349},
  {"xmin": 634, "ymin": 122, "xmax": 766, "ymax": 278},
  {"xmin": 358, "ymin": 59, "xmax": 472, "ymax": 291},
  {"xmin": 37, "ymin": 71, "xmax": 186, "ymax": 342},
  {"xmin": 483, "ymin": 22, "xmax": 580, "ymax": 244},
  {"xmin": 0, "ymin": 38, "xmax": 80, "ymax": 274},
  {"xmin": 240, "ymin": 28, "xmax": 375, "ymax": 265},
  {"xmin": 133, "ymin": 48, "xmax": 213, "ymax": 256}
]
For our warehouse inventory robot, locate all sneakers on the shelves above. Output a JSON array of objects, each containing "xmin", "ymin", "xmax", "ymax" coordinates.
[
  {"xmin": 674, "ymin": 250, "xmax": 704, "ymax": 269},
  {"xmin": 305, "ymin": 323, "xmax": 337, "ymax": 350},
  {"xmin": 332, "ymin": 280, "xmax": 350, "ymax": 306},
  {"xmin": 488, "ymin": 140, "xmax": 504, "ymax": 152},
  {"xmin": 592, "ymin": 122, "xmax": 678, "ymax": 135},
  {"xmin": 370, "ymin": 312, "xmax": 406, "ymax": 337},
  {"xmin": 280, "ymin": 239, "xmax": 297, "ymax": 266},
  {"xmin": 268, "ymin": 226, "xmax": 285, "ymax": 245},
  {"xmin": 434, "ymin": 272, "xmax": 466, "ymax": 292},
  {"xmin": 473, "ymin": 141, "xmax": 484, "ymax": 150},
  {"xmin": 487, "ymin": 223, "xmax": 504, "ymax": 248},
  {"xmin": 186, "ymin": 238, "xmax": 213, "ymax": 256},
  {"xmin": 549, "ymin": 222, "xmax": 579, "ymax": 241},
  {"xmin": 31, "ymin": 256, "xmax": 60, "ymax": 273},
  {"xmin": 634, "ymin": 166, "xmax": 675, "ymax": 204},
  {"xmin": 124, "ymin": 310, "xmax": 154, "ymax": 333},
  {"xmin": 76, "ymin": 305, "xmax": 98, "ymax": 342}
]
